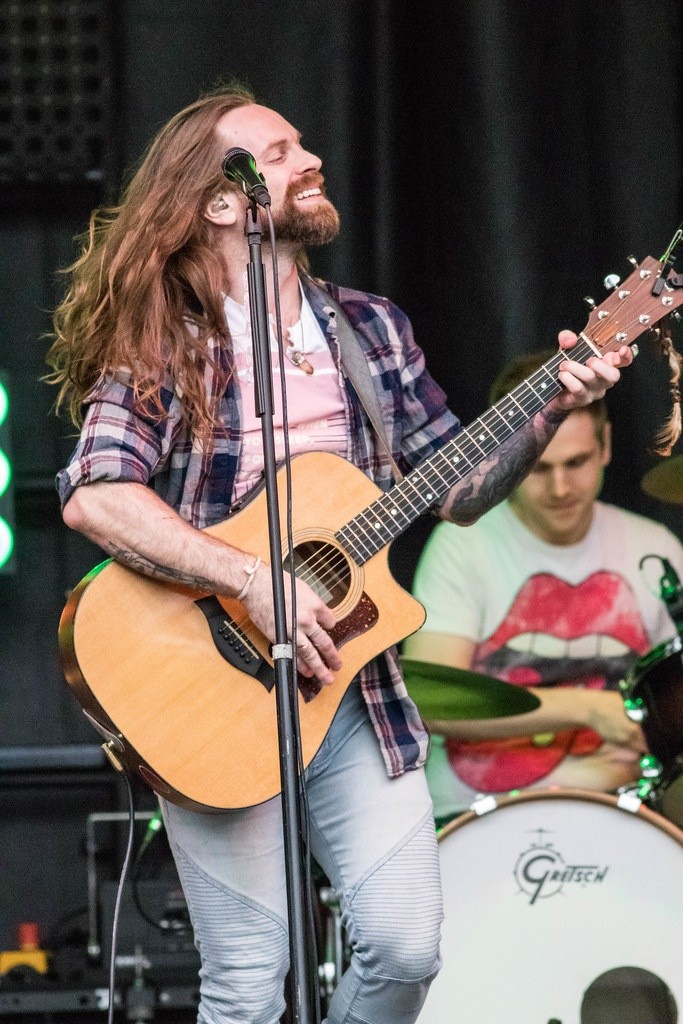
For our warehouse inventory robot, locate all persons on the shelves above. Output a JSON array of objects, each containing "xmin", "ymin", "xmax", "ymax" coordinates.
[
  {"xmin": 40, "ymin": 86, "xmax": 638, "ymax": 1024},
  {"xmin": 395, "ymin": 351, "xmax": 680, "ymax": 811}
]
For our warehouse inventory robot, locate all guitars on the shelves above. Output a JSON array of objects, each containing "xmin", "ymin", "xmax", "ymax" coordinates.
[{"xmin": 58, "ymin": 228, "xmax": 683, "ymax": 817}]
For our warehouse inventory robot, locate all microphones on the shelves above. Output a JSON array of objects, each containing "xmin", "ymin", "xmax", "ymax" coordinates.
[{"xmin": 221, "ymin": 147, "xmax": 271, "ymax": 208}]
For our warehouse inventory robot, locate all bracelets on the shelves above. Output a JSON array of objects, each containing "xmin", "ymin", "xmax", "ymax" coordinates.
[{"xmin": 235, "ymin": 553, "xmax": 269, "ymax": 602}]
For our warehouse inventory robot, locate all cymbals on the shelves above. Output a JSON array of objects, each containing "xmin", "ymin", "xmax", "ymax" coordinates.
[{"xmin": 396, "ymin": 654, "xmax": 546, "ymax": 722}]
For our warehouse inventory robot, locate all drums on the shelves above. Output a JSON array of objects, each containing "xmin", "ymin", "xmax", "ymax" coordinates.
[
  {"xmin": 413, "ymin": 785, "xmax": 681, "ymax": 1024},
  {"xmin": 621, "ymin": 625, "xmax": 683, "ymax": 774}
]
